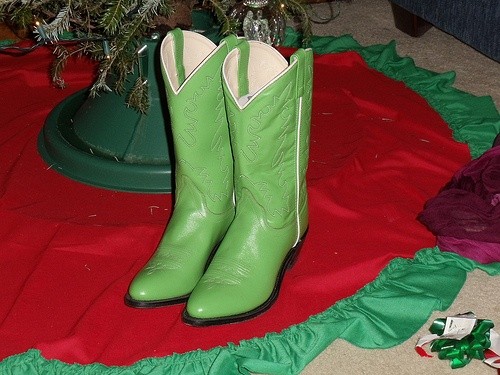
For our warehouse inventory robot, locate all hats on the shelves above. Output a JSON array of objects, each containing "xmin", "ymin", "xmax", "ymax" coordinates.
[{"xmin": 37, "ymin": 25, "xmax": 177, "ymax": 193}]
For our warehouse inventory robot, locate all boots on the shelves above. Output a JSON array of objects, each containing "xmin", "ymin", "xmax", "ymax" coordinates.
[
  {"xmin": 122, "ymin": 29, "xmax": 245, "ymax": 309},
  {"xmin": 182, "ymin": 39, "xmax": 314, "ymax": 326}
]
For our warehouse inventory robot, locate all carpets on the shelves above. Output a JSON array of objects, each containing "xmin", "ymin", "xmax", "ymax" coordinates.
[{"xmin": 0, "ymin": 27, "xmax": 500, "ymax": 374}]
